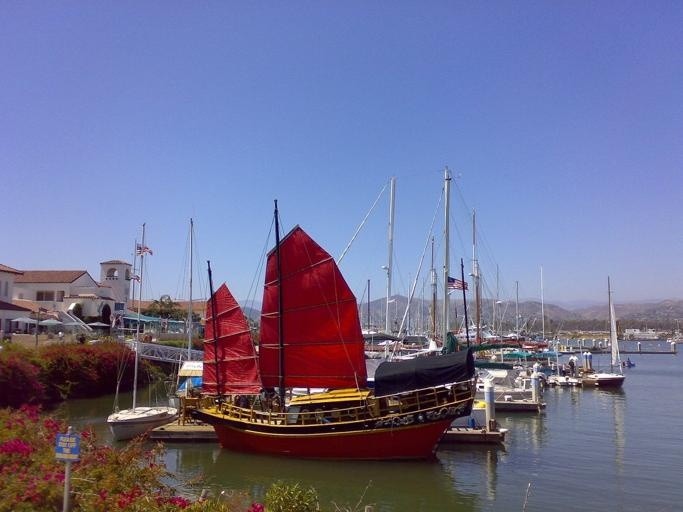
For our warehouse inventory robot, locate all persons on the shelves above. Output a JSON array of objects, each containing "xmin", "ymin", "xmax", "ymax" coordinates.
[
  {"xmin": 110, "ymin": 314, "xmax": 122, "ymax": 337},
  {"xmin": 233, "ymin": 393, "xmax": 280, "ymax": 417},
  {"xmin": 319, "ymin": 415, "xmax": 329, "ymax": 423},
  {"xmin": 568, "ymin": 353, "xmax": 579, "ymax": 379}
]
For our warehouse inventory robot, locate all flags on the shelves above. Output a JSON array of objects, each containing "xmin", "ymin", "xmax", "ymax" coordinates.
[
  {"xmin": 133, "ymin": 242, "xmax": 153, "ymax": 258},
  {"xmin": 445, "ymin": 275, "xmax": 468, "ymax": 291},
  {"xmin": 126, "ymin": 270, "xmax": 140, "ymax": 283}
]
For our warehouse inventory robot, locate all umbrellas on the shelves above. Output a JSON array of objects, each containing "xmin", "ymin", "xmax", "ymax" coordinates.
[{"xmin": 9, "ymin": 316, "xmax": 110, "ymax": 338}]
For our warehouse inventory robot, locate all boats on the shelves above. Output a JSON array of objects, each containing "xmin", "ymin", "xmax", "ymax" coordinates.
[
  {"xmin": 622, "ymin": 317, "xmax": 661, "ymax": 340},
  {"xmin": 625, "ymin": 360, "xmax": 636, "ymax": 367},
  {"xmin": 666, "ymin": 335, "xmax": 683, "ymax": 344}
]
